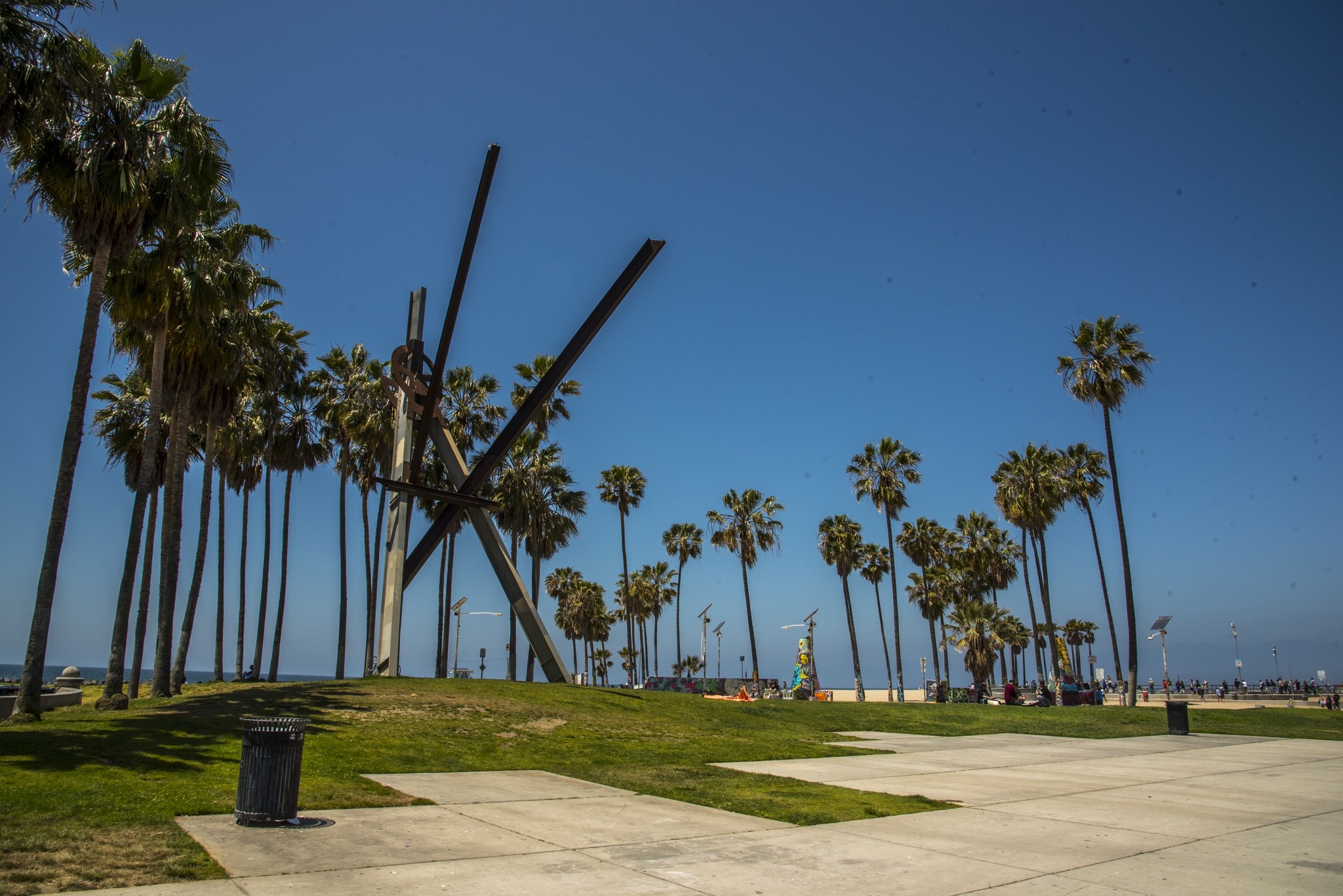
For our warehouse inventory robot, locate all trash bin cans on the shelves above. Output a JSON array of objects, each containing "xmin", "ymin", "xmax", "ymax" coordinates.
[
  {"xmin": 1164, "ymin": 700, "xmax": 1190, "ymax": 735},
  {"xmin": 232, "ymin": 714, "xmax": 312, "ymax": 826}
]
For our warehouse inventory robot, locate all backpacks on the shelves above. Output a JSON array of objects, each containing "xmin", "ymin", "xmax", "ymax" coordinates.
[{"xmin": 1149, "ymin": 681, "xmax": 1153, "ymax": 685}]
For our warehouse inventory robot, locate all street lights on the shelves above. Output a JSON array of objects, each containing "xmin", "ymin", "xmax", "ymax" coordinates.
[
  {"xmin": 695, "ymin": 603, "xmax": 711, "ymax": 696},
  {"xmin": 782, "ymin": 608, "xmax": 819, "ymax": 699},
  {"xmin": 1148, "ymin": 616, "xmax": 1170, "ymax": 699},
  {"xmin": 712, "ymin": 621, "xmax": 727, "ymax": 686},
  {"xmin": 1231, "ymin": 623, "xmax": 1243, "ymax": 692},
  {"xmin": 1270, "ymin": 644, "xmax": 1280, "ymax": 682},
  {"xmin": 451, "ymin": 596, "xmax": 502, "ymax": 678}
]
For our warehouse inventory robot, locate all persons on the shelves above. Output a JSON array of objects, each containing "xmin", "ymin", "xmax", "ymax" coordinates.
[
  {"xmin": 734, "ymin": 685, "xmax": 750, "ymax": 700},
  {"xmin": 752, "ymin": 683, "xmax": 756, "ymax": 698},
  {"xmin": 917, "ymin": 677, "xmax": 1343, "ymax": 711},
  {"xmin": 242, "ymin": 664, "xmax": 253, "ymax": 680}
]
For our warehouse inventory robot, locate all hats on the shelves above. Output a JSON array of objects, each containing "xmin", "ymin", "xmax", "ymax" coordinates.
[
  {"xmin": 1220, "ymin": 687, "xmax": 1223, "ymax": 689},
  {"xmin": 981, "ymin": 682, "xmax": 987, "ymax": 686},
  {"xmin": 1149, "ymin": 678, "xmax": 1152, "ymax": 680},
  {"xmin": 1014, "ymin": 683, "xmax": 1019, "ymax": 686}
]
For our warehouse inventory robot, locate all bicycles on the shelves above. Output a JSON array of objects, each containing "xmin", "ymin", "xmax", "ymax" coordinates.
[{"xmin": 366, "ymin": 656, "xmax": 402, "ymax": 676}]
